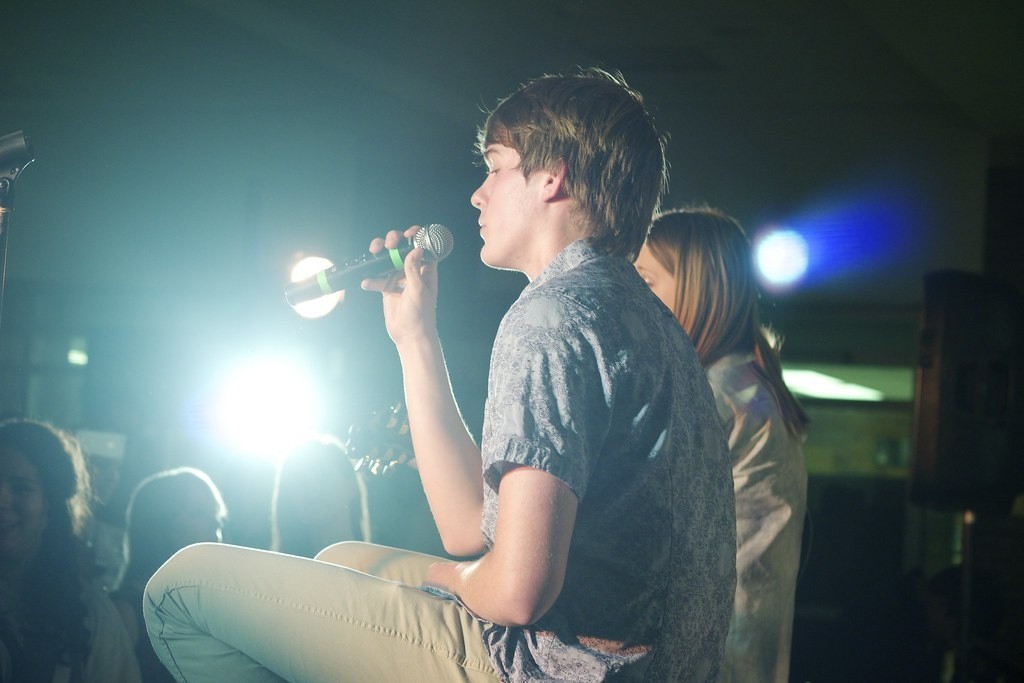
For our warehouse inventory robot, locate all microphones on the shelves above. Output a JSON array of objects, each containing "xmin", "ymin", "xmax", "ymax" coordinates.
[{"xmin": 287, "ymin": 224, "xmax": 455, "ymax": 306}]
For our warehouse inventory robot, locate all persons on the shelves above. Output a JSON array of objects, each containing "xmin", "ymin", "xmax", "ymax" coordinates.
[
  {"xmin": 0, "ymin": 420, "xmax": 98, "ymax": 683},
  {"xmin": 269, "ymin": 436, "xmax": 367, "ymax": 558},
  {"xmin": 141, "ymin": 69, "xmax": 738, "ymax": 682},
  {"xmin": 633, "ymin": 204, "xmax": 805, "ymax": 682},
  {"xmin": 109, "ymin": 468, "xmax": 226, "ymax": 683}
]
